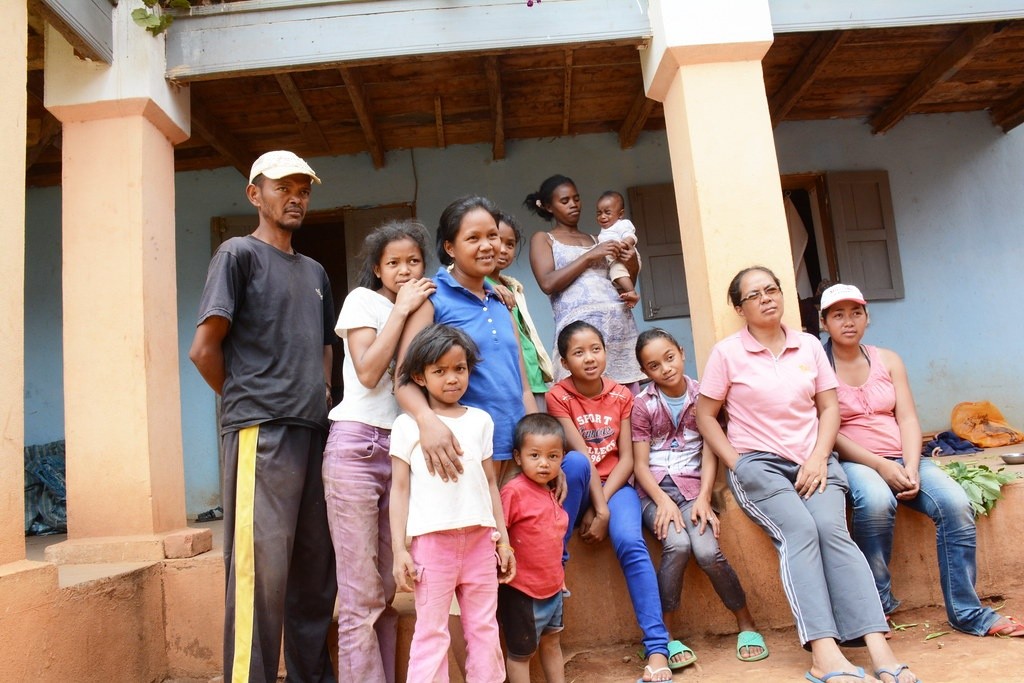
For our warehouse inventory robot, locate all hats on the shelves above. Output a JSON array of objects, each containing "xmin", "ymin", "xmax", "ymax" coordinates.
[
  {"xmin": 820, "ymin": 283, "xmax": 865, "ymax": 313},
  {"xmin": 249, "ymin": 150, "xmax": 321, "ymax": 185}
]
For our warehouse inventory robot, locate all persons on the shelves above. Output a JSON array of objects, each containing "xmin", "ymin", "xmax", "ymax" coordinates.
[
  {"xmin": 323, "ymin": 222, "xmax": 436, "ymax": 683},
  {"xmin": 695, "ymin": 267, "xmax": 919, "ymax": 683},
  {"xmin": 544, "ymin": 319, "xmax": 673, "ymax": 683},
  {"xmin": 524, "ymin": 171, "xmax": 648, "ymax": 398},
  {"xmin": 595, "ymin": 191, "xmax": 642, "ymax": 309},
  {"xmin": 818, "ymin": 283, "xmax": 1023, "ymax": 639},
  {"xmin": 393, "ymin": 191, "xmax": 538, "ymax": 490},
  {"xmin": 448, "ymin": 210, "xmax": 554, "ymax": 412},
  {"xmin": 629, "ymin": 325, "xmax": 770, "ymax": 668},
  {"xmin": 189, "ymin": 150, "xmax": 338, "ymax": 683},
  {"xmin": 498, "ymin": 412, "xmax": 570, "ymax": 683},
  {"xmin": 387, "ymin": 323, "xmax": 516, "ymax": 683}
]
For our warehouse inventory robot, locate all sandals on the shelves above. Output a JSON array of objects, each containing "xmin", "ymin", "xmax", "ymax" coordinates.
[{"xmin": 194, "ymin": 506, "xmax": 224, "ymax": 521}]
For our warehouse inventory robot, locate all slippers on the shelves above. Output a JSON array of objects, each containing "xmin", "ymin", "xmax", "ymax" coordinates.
[
  {"xmin": 637, "ymin": 665, "xmax": 672, "ymax": 683},
  {"xmin": 986, "ymin": 616, "xmax": 1024, "ymax": 636},
  {"xmin": 562, "ymin": 587, "xmax": 571, "ymax": 598},
  {"xmin": 736, "ymin": 631, "xmax": 768, "ymax": 661},
  {"xmin": 667, "ymin": 640, "xmax": 697, "ymax": 669},
  {"xmin": 875, "ymin": 664, "xmax": 922, "ymax": 683},
  {"xmin": 805, "ymin": 666, "xmax": 865, "ymax": 683},
  {"xmin": 883, "ymin": 614, "xmax": 892, "ymax": 639}
]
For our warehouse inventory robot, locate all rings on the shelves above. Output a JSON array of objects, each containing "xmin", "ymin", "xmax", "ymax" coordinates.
[{"xmin": 814, "ymin": 480, "xmax": 819, "ymax": 484}]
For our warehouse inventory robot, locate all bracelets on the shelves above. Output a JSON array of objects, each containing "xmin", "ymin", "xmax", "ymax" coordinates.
[{"xmin": 496, "ymin": 544, "xmax": 514, "ymax": 554}]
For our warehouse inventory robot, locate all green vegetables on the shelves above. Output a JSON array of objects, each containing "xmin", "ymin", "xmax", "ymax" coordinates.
[{"xmin": 933, "ymin": 460, "xmax": 1018, "ymax": 520}]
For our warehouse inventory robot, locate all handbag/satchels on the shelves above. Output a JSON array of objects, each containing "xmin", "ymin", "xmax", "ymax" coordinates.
[{"xmin": 951, "ymin": 400, "xmax": 1024, "ymax": 448}]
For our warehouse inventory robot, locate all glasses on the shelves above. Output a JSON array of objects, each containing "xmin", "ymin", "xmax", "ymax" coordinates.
[{"xmin": 739, "ymin": 286, "xmax": 781, "ymax": 305}]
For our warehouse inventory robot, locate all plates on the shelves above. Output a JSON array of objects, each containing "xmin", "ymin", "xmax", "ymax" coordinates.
[{"xmin": 999, "ymin": 453, "xmax": 1024, "ymax": 465}]
